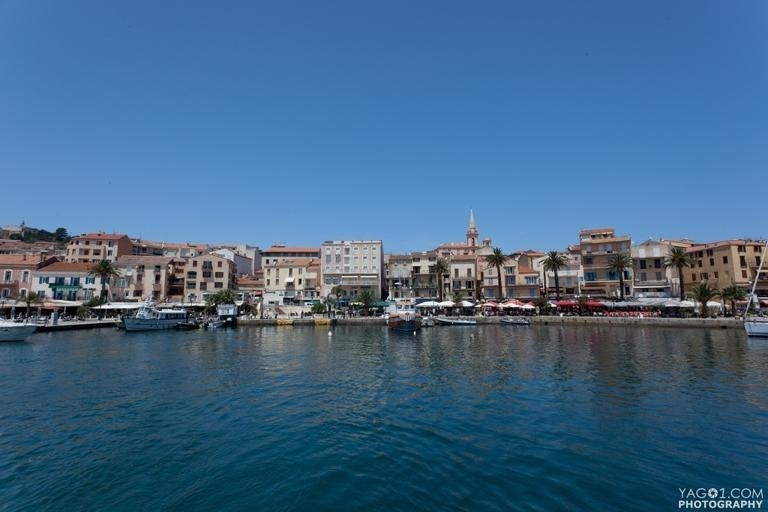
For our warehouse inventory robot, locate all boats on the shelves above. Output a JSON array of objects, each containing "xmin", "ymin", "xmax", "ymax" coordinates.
[
  {"xmin": 388, "ymin": 313, "xmax": 478, "ymax": 331},
  {"xmin": 501, "ymin": 319, "xmax": 530, "ymax": 325},
  {"xmin": 114, "ymin": 297, "xmax": 232, "ymax": 330},
  {"xmin": 1, "ymin": 318, "xmax": 37, "ymax": 342}
]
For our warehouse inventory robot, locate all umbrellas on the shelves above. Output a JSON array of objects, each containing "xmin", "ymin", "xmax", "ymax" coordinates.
[{"xmin": 416, "ymin": 297, "xmax": 558, "ymax": 315}]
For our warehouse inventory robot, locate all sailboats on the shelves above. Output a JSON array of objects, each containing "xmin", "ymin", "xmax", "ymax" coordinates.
[{"xmin": 743, "ymin": 242, "xmax": 768, "ymax": 337}]
